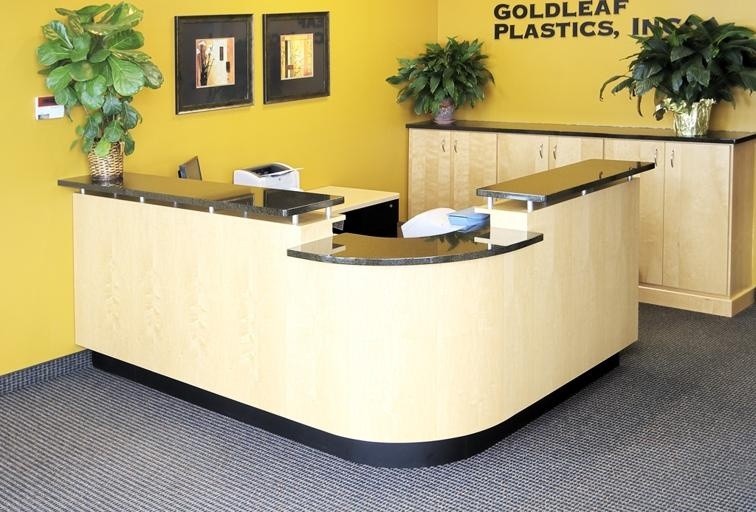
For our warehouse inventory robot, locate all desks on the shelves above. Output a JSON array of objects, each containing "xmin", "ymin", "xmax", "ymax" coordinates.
[{"xmin": 305, "ymin": 185, "xmax": 401, "ymax": 238}]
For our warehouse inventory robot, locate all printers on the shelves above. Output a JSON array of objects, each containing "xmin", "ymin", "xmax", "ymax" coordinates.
[{"xmin": 233, "ymin": 162, "xmax": 300, "ymax": 192}]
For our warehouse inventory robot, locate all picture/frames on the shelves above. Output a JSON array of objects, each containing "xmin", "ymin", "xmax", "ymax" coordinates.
[{"xmin": 173, "ymin": 9, "xmax": 331, "ymax": 115}]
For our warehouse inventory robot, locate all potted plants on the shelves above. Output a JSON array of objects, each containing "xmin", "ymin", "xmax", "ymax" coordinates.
[
  {"xmin": 34, "ymin": 0, "xmax": 164, "ymax": 183},
  {"xmin": 385, "ymin": 36, "xmax": 497, "ymax": 126},
  {"xmin": 599, "ymin": 14, "xmax": 756, "ymax": 139}
]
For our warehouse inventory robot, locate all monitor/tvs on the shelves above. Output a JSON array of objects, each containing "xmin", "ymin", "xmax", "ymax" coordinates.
[{"xmin": 180, "ymin": 155, "xmax": 202, "ymax": 180}]
